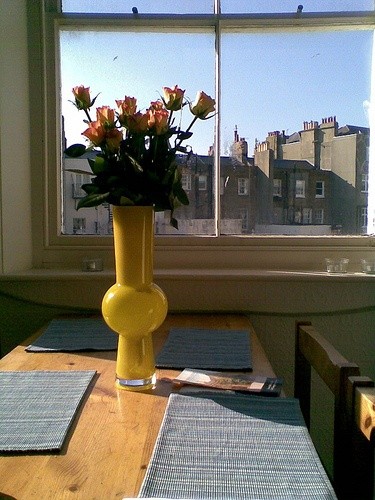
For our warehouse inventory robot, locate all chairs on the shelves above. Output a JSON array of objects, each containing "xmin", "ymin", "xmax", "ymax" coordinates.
[
  {"xmin": 295, "ymin": 321, "xmax": 360, "ymax": 490},
  {"xmin": 338, "ymin": 375, "xmax": 374, "ymax": 500}
]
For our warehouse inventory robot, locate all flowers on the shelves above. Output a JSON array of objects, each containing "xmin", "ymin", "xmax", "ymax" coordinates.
[{"xmin": 64, "ymin": 84, "xmax": 218, "ymax": 231}]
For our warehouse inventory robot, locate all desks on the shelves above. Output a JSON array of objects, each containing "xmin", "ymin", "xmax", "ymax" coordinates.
[{"xmin": 0, "ymin": 313, "xmax": 288, "ymax": 500}]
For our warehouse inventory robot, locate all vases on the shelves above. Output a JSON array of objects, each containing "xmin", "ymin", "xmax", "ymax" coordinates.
[{"xmin": 102, "ymin": 206, "xmax": 169, "ymax": 392}]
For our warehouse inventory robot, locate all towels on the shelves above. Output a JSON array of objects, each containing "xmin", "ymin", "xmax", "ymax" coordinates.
[
  {"xmin": 25, "ymin": 319, "xmax": 119, "ymax": 353},
  {"xmin": 155, "ymin": 328, "xmax": 254, "ymax": 372},
  {"xmin": 0, "ymin": 371, "xmax": 98, "ymax": 452},
  {"xmin": 137, "ymin": 392, "xmax": 335, "ymax": 499}
]
